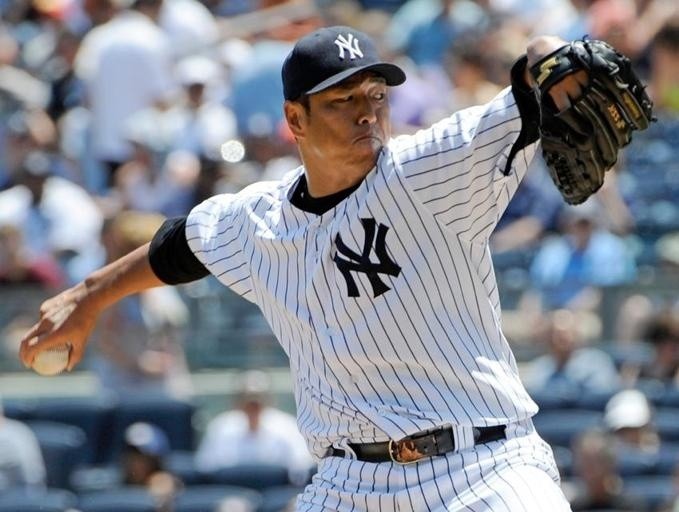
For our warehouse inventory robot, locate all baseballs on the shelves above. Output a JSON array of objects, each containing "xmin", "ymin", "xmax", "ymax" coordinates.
[{"xmin": 27, "ymin": 335, "xmax": 69, "ymax": 376}]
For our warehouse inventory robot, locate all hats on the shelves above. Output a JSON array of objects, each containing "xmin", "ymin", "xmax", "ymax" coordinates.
[{"xmin": 279, "ymin": 24, "xmax": 406, "ymax": 97}]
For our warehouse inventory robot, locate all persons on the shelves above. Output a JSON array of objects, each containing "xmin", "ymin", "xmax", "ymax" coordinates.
[{"xmin": 1, "ymin": 0, "xmax": 679, "ymax": 512}]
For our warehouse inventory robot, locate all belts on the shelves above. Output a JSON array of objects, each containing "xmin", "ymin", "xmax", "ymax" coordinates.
[{"xmin": 328, "ymin": 425, "xmax": 509, "ymax": 466}]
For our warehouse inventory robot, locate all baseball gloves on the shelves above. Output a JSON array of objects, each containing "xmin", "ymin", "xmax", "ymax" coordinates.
[{"xmin": 529, "ymin": 39, "xmax": 653, "ymax": 206}]
